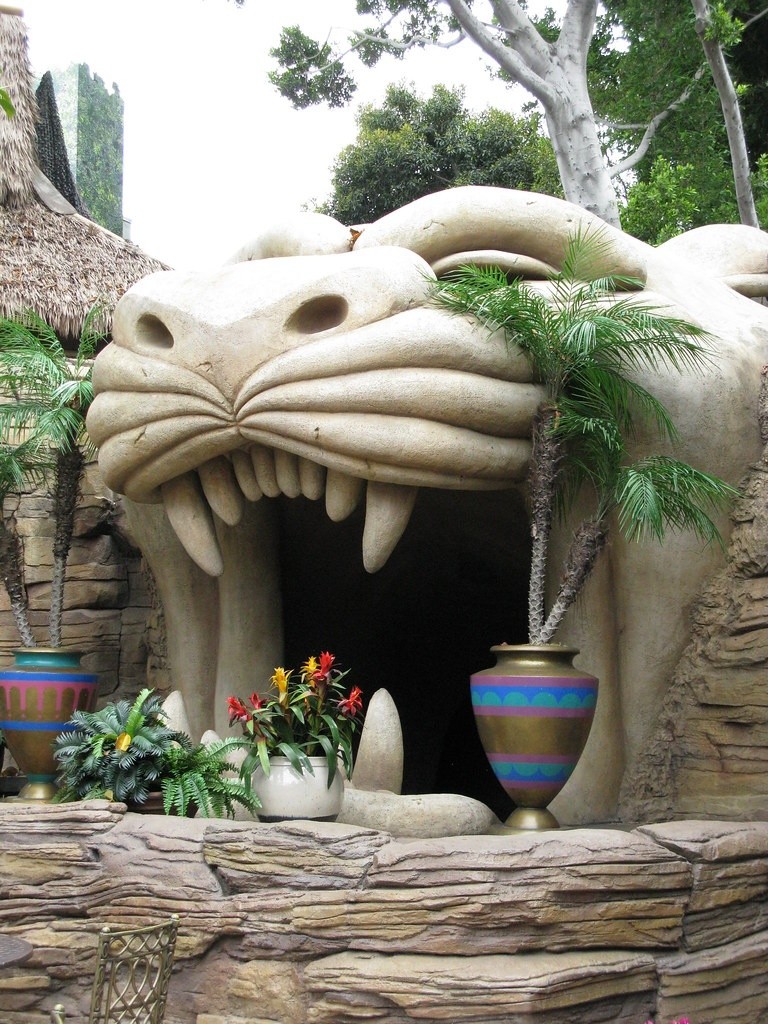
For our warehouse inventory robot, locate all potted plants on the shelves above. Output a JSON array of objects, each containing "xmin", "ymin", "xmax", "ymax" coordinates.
[
  {"xmin": 415, "ymin": 210, "xmax": 753, "ymax": 837},
  {"xmin": 50, "ymin": 686, "xmax": 263, "ymax": 821},
  {"xmin": 222, "ymin": 650, "xmax": 365, "ymax": 822},
  {"xmin": 0, "ymin": 294, "xmax": 110, "ymax": 803}
]
